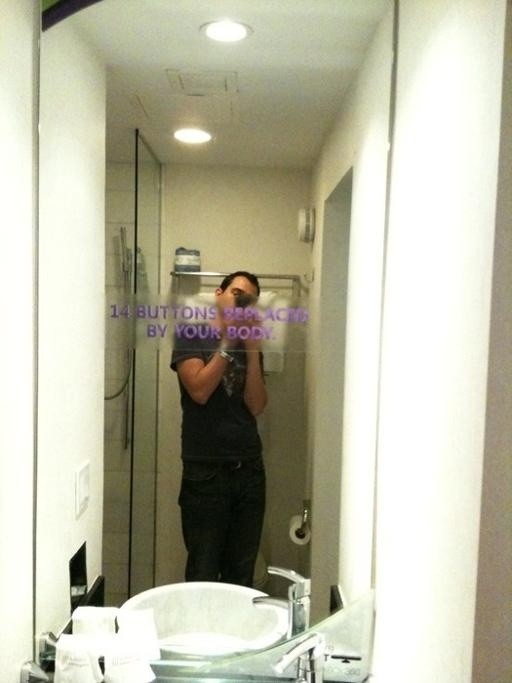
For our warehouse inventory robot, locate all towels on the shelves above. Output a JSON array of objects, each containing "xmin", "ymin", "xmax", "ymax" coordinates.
[
  {"xmin": 54, "ymin": 635, "xmax": 101, "ymax": 682},
  {"xmin": 185, "ymin": 294, "xmax": 289, "ymax": 371},
  {"xmin": 103, "ymin": 637, "xmax": 158, "ymax": 682},
  {"xmin": 118, "ymin": 609, "xmax": 161, "ymax": 661},
  {"xmin": 71, "ymin": 603, "xmax": 118, "ymax": 632}
]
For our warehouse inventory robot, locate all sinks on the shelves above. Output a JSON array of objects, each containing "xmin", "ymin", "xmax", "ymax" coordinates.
[{"xmin": 118, "ymin": 581, "xmax": 289, "ymax": 658}]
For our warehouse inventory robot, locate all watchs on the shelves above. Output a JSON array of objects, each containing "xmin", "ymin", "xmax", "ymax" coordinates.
[{"xmin": 216, "ymin": 349, "xmax": 234, "ymax": 364}]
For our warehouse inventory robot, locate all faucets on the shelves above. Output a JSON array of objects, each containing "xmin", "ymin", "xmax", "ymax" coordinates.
[{"xmin": 251, "ymin": 564, "xmax": 312, "ymax": 642}]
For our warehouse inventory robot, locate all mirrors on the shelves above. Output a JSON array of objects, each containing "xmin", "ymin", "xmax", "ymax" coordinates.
[{"xmin": 33, "ymin": 0, "xmax": 399, "ymax": 683}]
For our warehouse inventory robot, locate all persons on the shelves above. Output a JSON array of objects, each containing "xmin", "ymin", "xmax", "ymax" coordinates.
[{"xmin": 171, "ymin": 271, "xmax": 267, "ymax": 588}]
